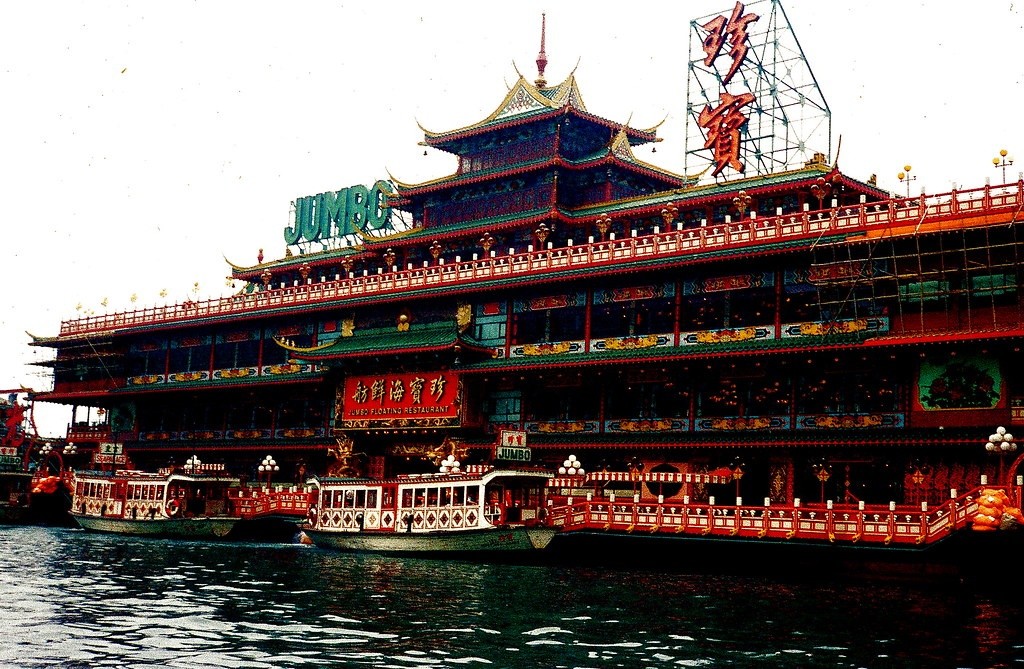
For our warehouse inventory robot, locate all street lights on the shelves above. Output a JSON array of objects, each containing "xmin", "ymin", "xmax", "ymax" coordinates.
[
  {"xmin": 260, "ymin": 268, "xmax": 272, "ymax": 298},
  {"xmin": 733, "ymin": 189, "xmax": 752, "ymax": 221},
  {"xmin": 429, "ymin": 240, "xmax": 442, "ymax": 273},
  {"xmin": 62, "ymin": 442, "xmax": 78, "ymax": 472},
  {"xmin": 627, "ymin": 457, "xmax": 643, "ymax": 495},
  {"xmin": 299, "ymin": 262, "xmax": 312, "ymax": 292},
  {"xmin": 661, "ymin": 201, "xmax": 678, "ymax": 240},
  {"xmin": 257, "ymin": 455, "xmax": 280, "ymax": 489},
  {"xmin": 595, "ymin": 212, "xmax": 612, "ymax": 249},
  {"xmin": 340, "ymin": 255, "xmax": 354, "ymax": 286},
  {"xmin": 382, "ymin": 248, "xmax": 396, "ymax": 280},
  {"xmin": 812, "ymin": 457, "xmax": 833, "ymax": 503},
  {"xmin": 729, "ymin": 456, "xmax": 746, "ymax": 497},
  {"xmin": 897, "ymin": 164, "xmax": 917, "ymax": 208},
  {"xmin": 810, "ymin": 176, "xmax": 832, "ymax": 210},
  {"xmin": 38, "ymin": 442, "xmax": 54, "ymax": 478},
  {"xmin": 535, "ymin": 222, "xmax": 550, "ymax": 251},
  {"xmin": 992, "ymin": 149, "xmax": 1015, "ymax": 194},
  {"xmin": 479, "ymin": 232, "xmax": 495, "ymax": 265},
  {"xmin": 558, "ymin": 454, "xmax": 585, "ymax": 497},
  {"xmin": 985, "ymin": 426, "xmax": 1019, "ymax": 487}
]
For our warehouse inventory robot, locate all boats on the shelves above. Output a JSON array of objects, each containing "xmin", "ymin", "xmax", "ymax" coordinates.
[
  {"xmin": 66, "ymin": 464, "xmax": 242, "ymax": 540},
  {"xmin": 294, "ymin": 468, "xmax": 562, "ymax": 558}
]
